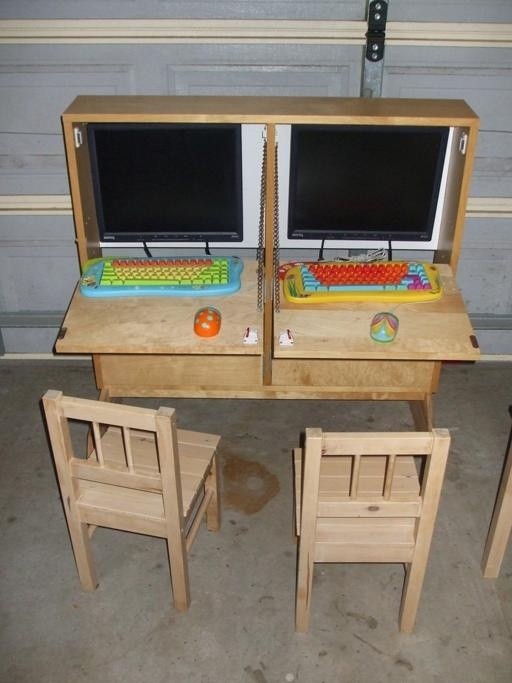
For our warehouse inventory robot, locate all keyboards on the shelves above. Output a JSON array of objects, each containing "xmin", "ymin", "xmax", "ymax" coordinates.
[
  {"xmin": 79, "ymin": 256, "xmax": 244, "ymax": 298},
  {"xmin": 283, "ymin": 261, "xmax": 443, "ymax": 304}
]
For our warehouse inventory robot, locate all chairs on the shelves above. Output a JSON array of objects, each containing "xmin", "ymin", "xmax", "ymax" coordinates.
[
  {"xmin": 42, "ymin": 389, "xmax": 222, "ymax": 613},
  {"xmin": 291, "ymin": 426, "xmax": 453, "ymax": 634}
]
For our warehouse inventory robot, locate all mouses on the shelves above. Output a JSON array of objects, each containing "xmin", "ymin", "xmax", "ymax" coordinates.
[
  {"xmin": 370, "ymin": 312, "xmax": 399, "ymax": 342},
  {"xmin": 194, "ymin": 307, "xmax": 221, "ymax": 337}
]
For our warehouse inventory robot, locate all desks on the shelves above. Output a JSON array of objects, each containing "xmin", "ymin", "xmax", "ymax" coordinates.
[{"xmin": 54, "ymin": 94, "xmax": 484, "ymax": 473}]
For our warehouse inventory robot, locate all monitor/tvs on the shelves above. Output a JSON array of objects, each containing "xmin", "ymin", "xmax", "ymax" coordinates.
[
  {"xmin": 288, "ymin": 124, "xmax": 450, "ymax": 241},
  {"xmin": 86, "ymin": 123, "xmax": 243, "ymax": 242}
]
